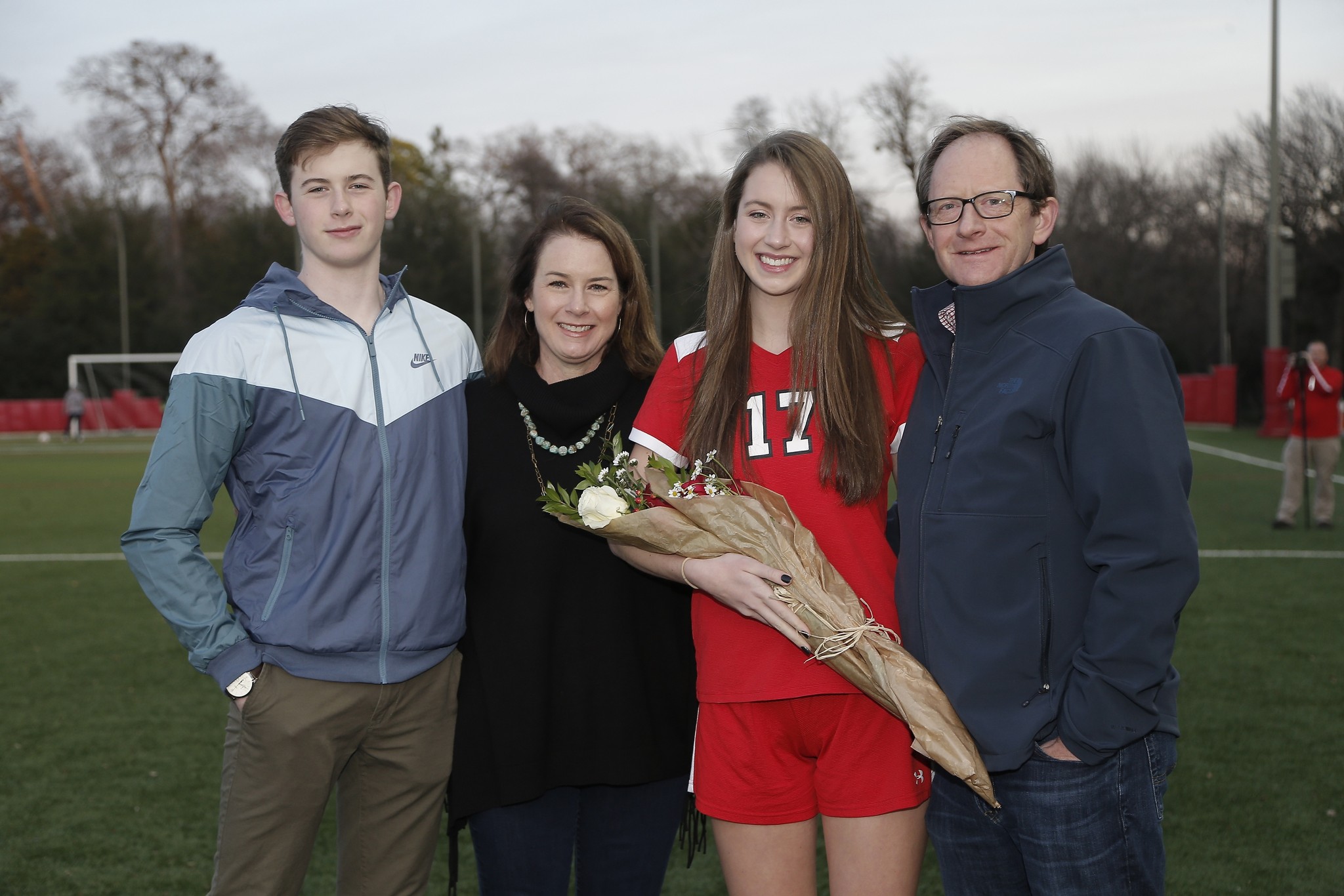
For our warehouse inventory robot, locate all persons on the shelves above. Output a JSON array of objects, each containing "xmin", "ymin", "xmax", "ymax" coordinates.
[
  {"xmin": 120, "ymin": 102, "xmax": 490, "ymax": 896},
  {"xmin": 62, "ymin": 381, "xmax": 86, "ymax": 439},
  {"xmin": 1270, "ymin": 337, "xmax": 1344, "ymax": 533},
  {"xmin": 878, "ymin": 111, "xmax": 1203, "ymax": 896},
  {"xmin": 607, "ymin": 117, "xmax": 940, "ymax": 896},
  {"xmin": 442, "ymin": 191, "xmax": 710, "ymax": 895}
]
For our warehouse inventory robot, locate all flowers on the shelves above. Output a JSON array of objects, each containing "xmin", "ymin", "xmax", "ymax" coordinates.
[{"xmin": 534, "ymin": 432, "xmax": 758, "ymax": 531}]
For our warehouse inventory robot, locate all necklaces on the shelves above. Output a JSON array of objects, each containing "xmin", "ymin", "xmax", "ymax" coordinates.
[{"xmin": 515, "ymin": 399, "xmax": 610, "ymax": 455}]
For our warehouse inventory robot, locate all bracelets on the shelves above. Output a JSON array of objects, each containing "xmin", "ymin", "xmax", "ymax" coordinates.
[
  {"xmin": 522, "ymin": 400, "xmax": 618, "ymax": 507},
  {"xmin": 681, "ymin": 555, "xmax": 699, "ymax": 589}
]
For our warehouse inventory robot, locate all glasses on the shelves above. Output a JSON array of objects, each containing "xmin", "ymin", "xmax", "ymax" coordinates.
[{"xmin": 920, "ymin": 189, "xmax": 1043, "ymax": 227}]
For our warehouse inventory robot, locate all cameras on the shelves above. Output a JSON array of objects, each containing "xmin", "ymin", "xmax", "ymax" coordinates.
[{"xmin": 1293, "ymin": 358, "xmax": 1307, "ymax": 370}]
